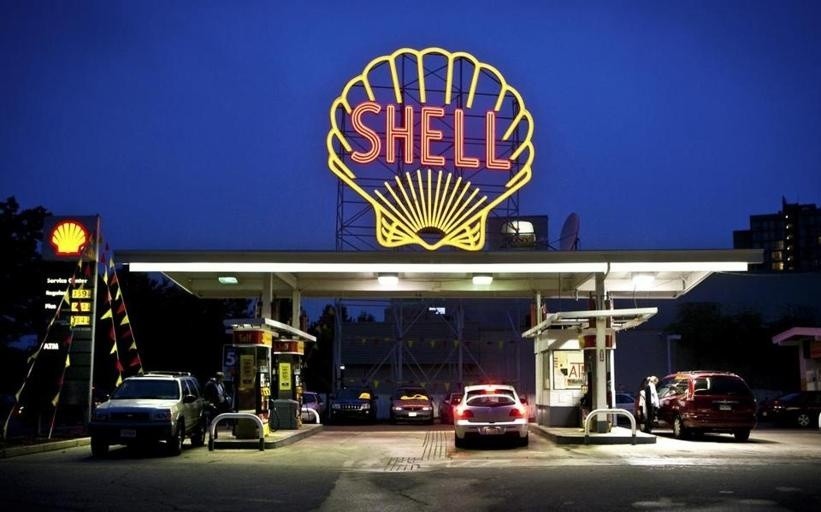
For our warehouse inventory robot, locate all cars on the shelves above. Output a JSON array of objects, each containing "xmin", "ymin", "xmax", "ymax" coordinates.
[
  {"xmin": 616, "ymin": 369, "xmax": 821, "ymax": 442},
  {"xmin": 438, "ymin": 384, "xmax": 529, "ymax": 445},
  {"xmin": 301, "ymin": 384, "xmax": 433, "ymax": 425},
  {"xmin": 89, "ymin": 370, "xmax": 209, "ymax": 456}
]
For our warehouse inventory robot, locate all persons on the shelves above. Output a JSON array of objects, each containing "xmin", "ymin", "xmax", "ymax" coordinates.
[
  {"xmin": 645, "ymin": 375, "xmax": 660, "ymax": 433},
  {"xmin": 639, "ymin": 379, "xmax": 646, "ymax": 431},
  {"xmin": 203, "ymin": 372, "xmax": 234, "ymax": 439}
]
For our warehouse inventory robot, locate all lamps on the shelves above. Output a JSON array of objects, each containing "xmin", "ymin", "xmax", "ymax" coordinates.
[
  {"xmin": 377, "ymin": 273, "xmax": 399, "ymax": 287},
  {"xmin": 472, "ymin": 273, "xmax": 493, "ymax": 285}
]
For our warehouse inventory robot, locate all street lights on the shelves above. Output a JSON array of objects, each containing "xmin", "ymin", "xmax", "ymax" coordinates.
[{"xmin": 334, "ymin": 362, "xmax": 345, "ymax": 392}]
